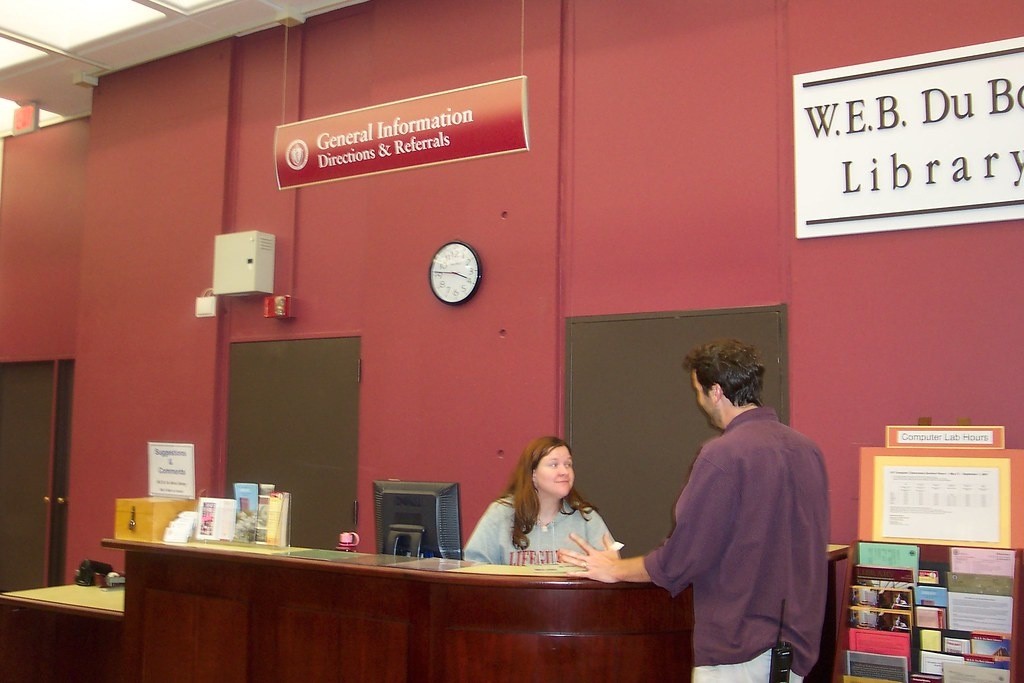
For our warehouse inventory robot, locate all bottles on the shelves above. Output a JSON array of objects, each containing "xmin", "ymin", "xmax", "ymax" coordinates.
[{"xmin": 336, "ymin": 531, "xmax": 359, "ymax": 552}]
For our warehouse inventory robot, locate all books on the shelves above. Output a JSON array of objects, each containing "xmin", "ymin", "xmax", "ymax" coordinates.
[{"xmin": 842, "ymin": 539, "xmax": 1016, "ymax": 683}]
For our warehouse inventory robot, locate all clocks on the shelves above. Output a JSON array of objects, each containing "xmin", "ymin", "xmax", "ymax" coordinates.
[{"xmin": 428, "ymin": 241, "xmax": 482, "ymax": 308}]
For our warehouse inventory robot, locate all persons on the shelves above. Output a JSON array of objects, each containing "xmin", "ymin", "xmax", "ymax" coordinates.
[
  {"xmin": 558, "ymin": 340, "xmax": 829, "ymax": 683},
  {"xmin": 463, "ymin": 436, "xmax": 622, "ymax": 565}
]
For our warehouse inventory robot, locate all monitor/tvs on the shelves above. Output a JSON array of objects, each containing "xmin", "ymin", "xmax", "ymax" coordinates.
[{"xmin": 371, "ymin": 480, "xmax": 463, "ymax": 561}]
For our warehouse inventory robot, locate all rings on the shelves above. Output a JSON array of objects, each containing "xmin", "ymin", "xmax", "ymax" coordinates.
[{"xmin": 583, "ymin": 562, "xmax": 587, "ymax": 568}]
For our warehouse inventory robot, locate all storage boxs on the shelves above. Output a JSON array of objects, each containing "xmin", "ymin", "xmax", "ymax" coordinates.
[{"xmin": 115, "ymin": 498, "xmax": 196, "ymax": 543}]
